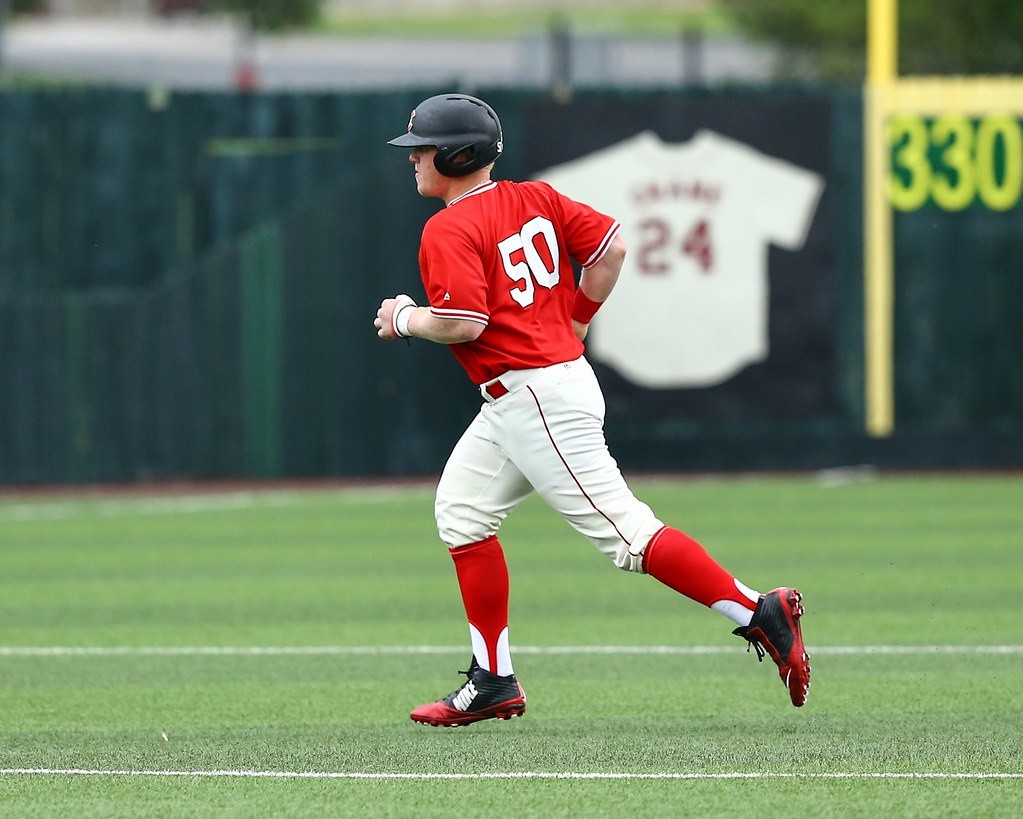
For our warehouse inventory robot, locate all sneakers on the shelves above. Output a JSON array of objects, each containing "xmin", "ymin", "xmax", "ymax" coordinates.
[
  {"xmin": 410, "ymin": 666, "xmax": 526, "ymax": 729},
  {"xmin": 732, "ymin": 587, "xmax": 812, "ymax": 707}
]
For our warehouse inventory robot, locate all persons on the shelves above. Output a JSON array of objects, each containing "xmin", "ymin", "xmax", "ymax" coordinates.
[{"xmin": 373, "ymin": 93, "xmax": 811, "ymax": 726}]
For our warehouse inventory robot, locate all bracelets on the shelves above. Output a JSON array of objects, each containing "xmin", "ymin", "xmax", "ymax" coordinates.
[{"xmin": 571, "ymin": 287, "xmax": 603, "ymax": 324}]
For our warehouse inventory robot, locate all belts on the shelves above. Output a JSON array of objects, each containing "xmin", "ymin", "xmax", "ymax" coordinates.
[{"xmin": 484, "ymin": 381, "xmax": 508, "ymax": 403}]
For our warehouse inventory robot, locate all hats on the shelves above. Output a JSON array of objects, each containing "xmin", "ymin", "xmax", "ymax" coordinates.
[{"xmin": 387, "ymin": 93, "xmax": 503, "ymax": 178}]
